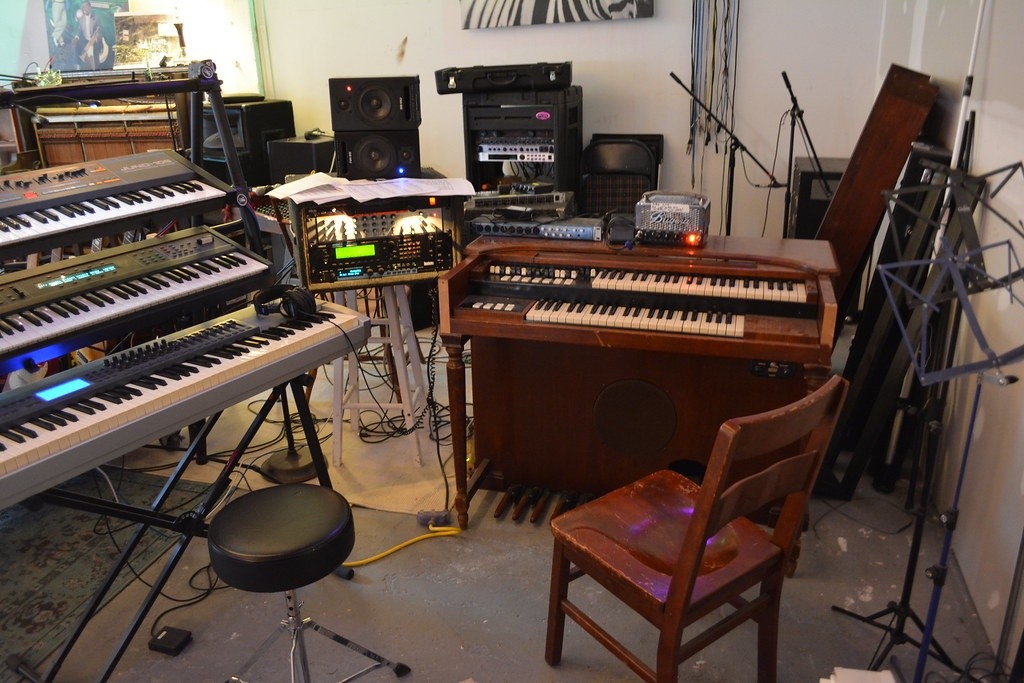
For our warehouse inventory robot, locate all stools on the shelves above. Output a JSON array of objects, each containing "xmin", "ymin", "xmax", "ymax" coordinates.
[{"xmin": 207, "ymin": 483, "xmax": 411, "ymax": 683}]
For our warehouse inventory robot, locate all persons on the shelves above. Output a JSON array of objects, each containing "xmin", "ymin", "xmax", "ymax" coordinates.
[
  {"xmin": 51, "ymin": 0, "xmax": 68, "ymax": 48},
  {"xmin": 70, "ymin": 0, "xmax": 103, "ymax": 70}
]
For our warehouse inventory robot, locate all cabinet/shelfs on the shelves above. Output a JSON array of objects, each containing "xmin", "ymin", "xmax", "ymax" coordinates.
[{"xmin": 25, "ymin": 66, "xmax": 190, "ymax": 168}]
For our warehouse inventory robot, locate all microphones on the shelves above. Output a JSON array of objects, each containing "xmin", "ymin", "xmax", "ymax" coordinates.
[{"xmin": 755, "ymin": 183, "xmax": 787, "ymax": 188}]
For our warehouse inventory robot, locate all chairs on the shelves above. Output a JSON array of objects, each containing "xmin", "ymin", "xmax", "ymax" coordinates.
[{"xmin": 545, "ymin": 374, "xmax": 852, "ymax": 683}]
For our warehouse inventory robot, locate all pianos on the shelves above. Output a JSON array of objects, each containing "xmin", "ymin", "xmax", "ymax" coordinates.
[
  {"xmin": 0, "ymin": 59, "xmax": 373, "ymax": 683},
  {"xmin": 428, "ymin": 224, "xmax": 839, "ymax": 530}
]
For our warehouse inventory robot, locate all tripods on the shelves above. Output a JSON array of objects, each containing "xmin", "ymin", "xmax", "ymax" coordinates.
[{"xmin": 832, "ymin": 270, "xmax": 984, "ymax": 683}]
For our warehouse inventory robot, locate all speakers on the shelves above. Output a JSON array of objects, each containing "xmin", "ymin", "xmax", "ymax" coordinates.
[
  {"xmin": 202, "ymin": 74, "xmax": 423, "ymax": 186},
  {"xmin": 788, "ymin": 157, "xmax": 851, "ymax": 239}
]
